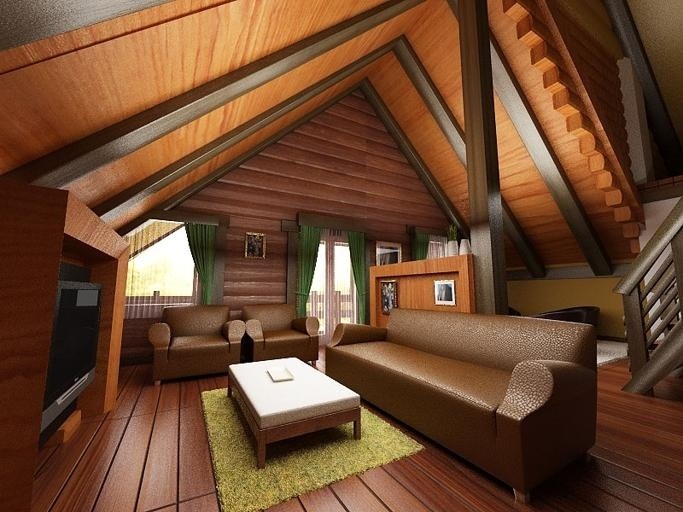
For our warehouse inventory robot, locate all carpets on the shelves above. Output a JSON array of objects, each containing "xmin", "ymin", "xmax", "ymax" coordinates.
[
  {"xmin": 201, "ymin": 387, "xmax": 424, "ymax": 512},
  {"xmin": 596, "ymin": 339, "xmax": 628, "ymax": 367}
]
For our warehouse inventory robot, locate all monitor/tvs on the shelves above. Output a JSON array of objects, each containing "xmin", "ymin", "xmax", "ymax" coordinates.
[{"xmin": 39, "ymin": 280, "xmax": 103, "ymax": 451}]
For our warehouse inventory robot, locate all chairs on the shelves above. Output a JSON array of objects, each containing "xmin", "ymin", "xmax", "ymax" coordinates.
[
  {"xmin": 241, "ymin": 305, "xmax": 319, "ymax": 368},
  {"xmin": 535, "ymin": 306, "xmax": 600, "ymax": 328},
  {"xmin": 148, "ymin": 305, "xmax": 246, "ymax": 386}
]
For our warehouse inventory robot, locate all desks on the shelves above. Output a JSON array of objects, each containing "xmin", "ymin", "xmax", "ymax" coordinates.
[{"xmin": 228, "ymin": 357, "xmax": 361, "ymax": 468}]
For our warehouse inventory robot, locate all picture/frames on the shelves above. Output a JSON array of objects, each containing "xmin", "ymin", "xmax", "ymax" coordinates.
[
  {"xmin": 376, "ymin": 240, "xmax": 402, "ymax": 266},
  {"xmin": 432, "ymin": 280, "xmax": 456, "ymax": 307},
  {"xmin": 380, "ymin": 281, "xmax": 398, "ymax": 315},
  {"xmin": 244, "ymin": 232, "xmax": 266, "ymax": 261}
]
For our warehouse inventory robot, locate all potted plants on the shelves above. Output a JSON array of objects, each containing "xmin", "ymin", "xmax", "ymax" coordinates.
[
  {"xmin": 459, "ymin": 224, "xmax": 472, "ymax": 255},
  {"xmin": 446, "ymin": 224, "xmax": 458, "ymax": 257}
]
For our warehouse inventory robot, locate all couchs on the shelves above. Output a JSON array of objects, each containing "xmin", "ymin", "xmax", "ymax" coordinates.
[{"xmin": 326, "ymin": 309, "xmax": 597, "ymax": 505}]
